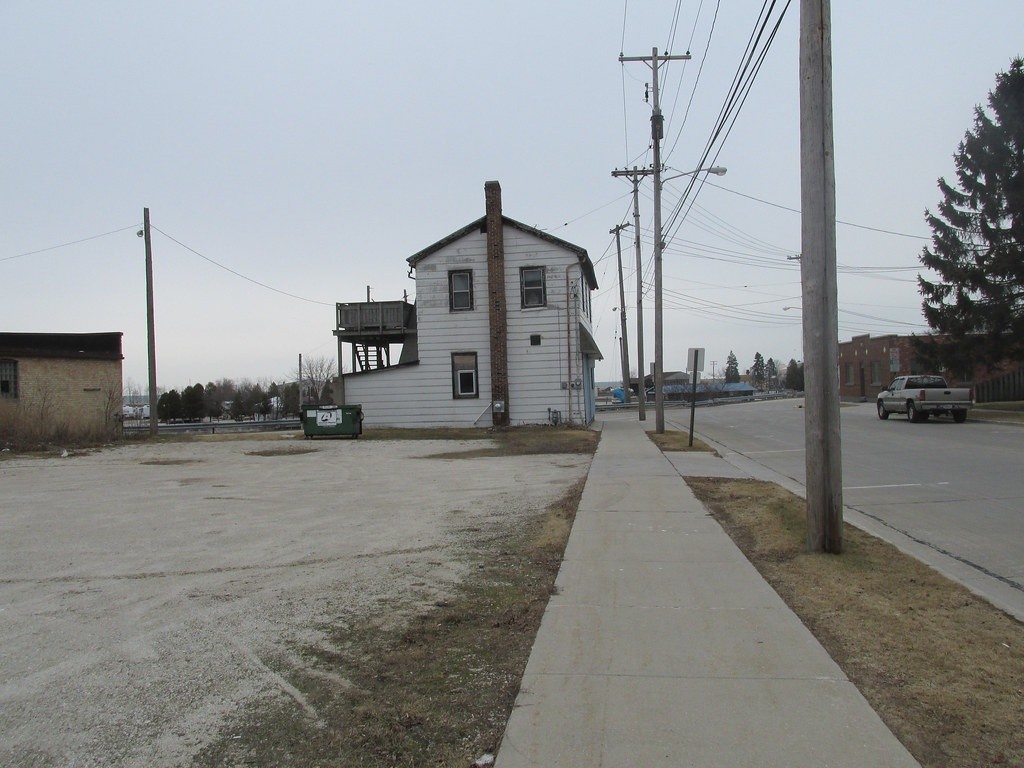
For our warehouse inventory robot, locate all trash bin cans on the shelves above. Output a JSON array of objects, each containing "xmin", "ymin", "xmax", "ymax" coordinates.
[{"xmin": 300, "ymin": 403, "xmax": 363, "ymax": 440}]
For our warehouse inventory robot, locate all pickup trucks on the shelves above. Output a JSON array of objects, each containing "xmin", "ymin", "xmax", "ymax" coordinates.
[{"xmin": 876, "ymin": 374, "xmax": 976, "ymax": 424}]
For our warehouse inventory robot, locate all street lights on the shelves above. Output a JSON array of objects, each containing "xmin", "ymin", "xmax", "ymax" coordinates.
[
  {"xmin": 611, "ymin": 307, "xmax": 632, "ymax": 409},
  {"xmin": 655, "ymin": 165, "xmax": 729, "ymax": 435}
]
